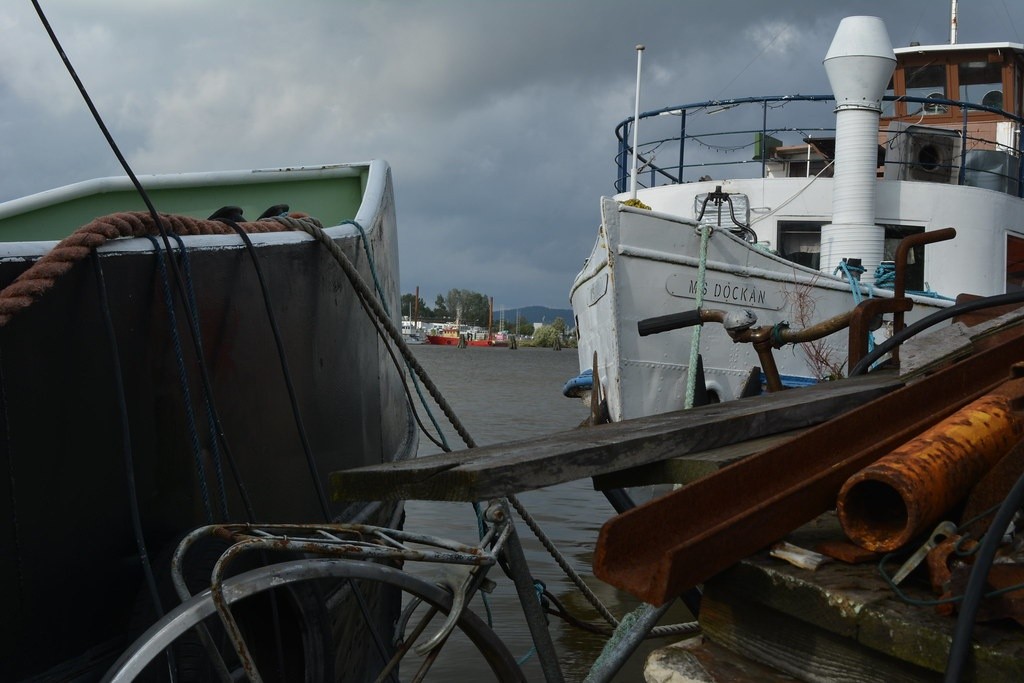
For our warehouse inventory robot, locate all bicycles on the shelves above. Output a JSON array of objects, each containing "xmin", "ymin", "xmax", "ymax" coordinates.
[{"xmin": 97, "ymin": 294, "xmax": 915, "ymax": 682}]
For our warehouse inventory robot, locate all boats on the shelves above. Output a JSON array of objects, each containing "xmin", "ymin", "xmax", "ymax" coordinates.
[
  {"xmin": 402, "ymin": 286, "xmax": 511, "ymax": 348},
  {"xmin": 568, "ymin": 0, "xmax": 1024, "ymax": 595}
]
